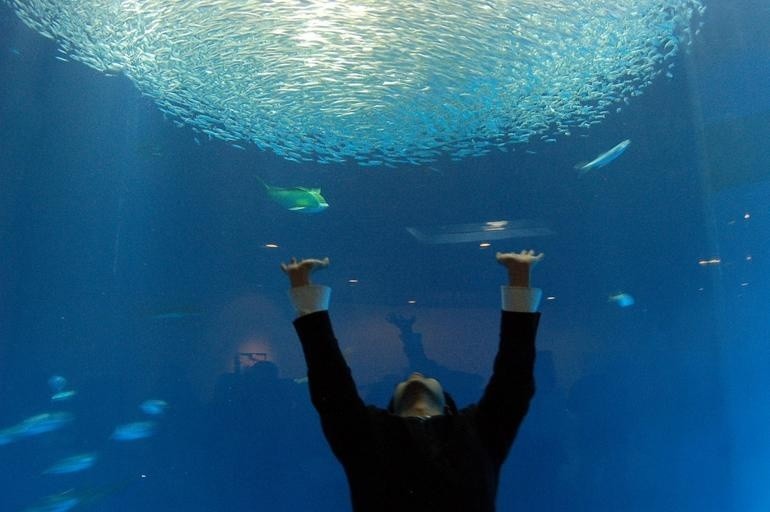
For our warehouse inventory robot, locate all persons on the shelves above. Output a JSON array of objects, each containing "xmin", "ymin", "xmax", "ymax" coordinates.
[
  {"xmin": 279, "ymin": 249, "xmax": 545, "ymax": 512},
  {"xmin": 380, "ymin": 304, "xmax": 486, "ymax": 380}
]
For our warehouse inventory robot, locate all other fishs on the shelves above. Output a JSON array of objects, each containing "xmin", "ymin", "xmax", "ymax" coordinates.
[{"xmin": 0, "ymin": 0, "xmax": 713, "ymax": 171}]
